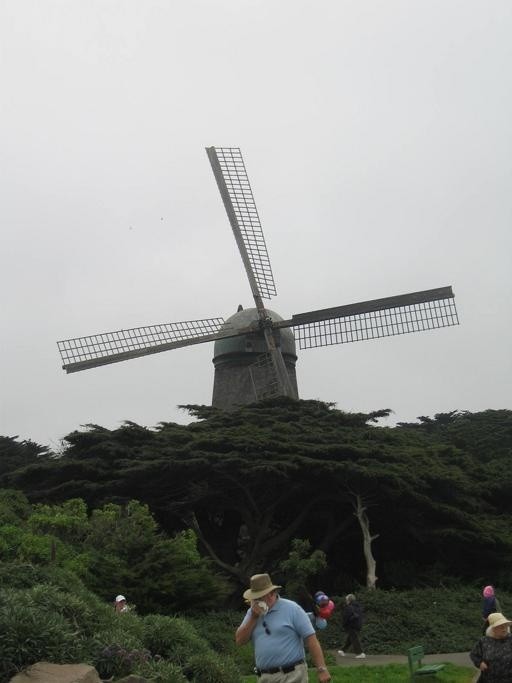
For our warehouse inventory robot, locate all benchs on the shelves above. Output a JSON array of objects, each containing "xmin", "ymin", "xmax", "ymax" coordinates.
[{"xmin": 408, "ymin": 645, "xmax": 445, "ymax": 678}]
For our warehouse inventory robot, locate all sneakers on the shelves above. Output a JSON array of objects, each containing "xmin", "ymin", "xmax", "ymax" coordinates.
[
  {"xmin": 338, "ymin": 651, "xmax": 345, "ymax": 656},
  {"xmin": 352, "ymin": 653, "xmax": 366, "ymax": 659}
]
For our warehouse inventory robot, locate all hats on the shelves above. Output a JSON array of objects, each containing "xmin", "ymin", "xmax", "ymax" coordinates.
[
  {"xmin": 116, "ymin": 595, "xmax": 126, "ymax": 602},
  {"xmin": 488, "ymin": 613, "xmax": 512, "ymax": 628},
  {"xmin": 243, "ymin": 573, "xmax": 282, "ymax": 600},
  {"xmin": 484, "ymin": 586, "xmax": 494, "ymax": 597}
]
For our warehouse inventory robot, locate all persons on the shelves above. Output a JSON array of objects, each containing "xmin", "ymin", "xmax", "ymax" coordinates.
[
  {"xmin": 336, "ymin": 593, "xmax": 368, "ymax": 660},
  {"xmin": 233, "ymin": 571, "xmax": 333, "ymax": 683},
  {"xmin": 468, "ymin": 611, "xmax": 511, "ymax": 682},
  {"xmin": 480, "ymin": 584, "xmax": 497, "ymax": 637},
  {"xmin": 114, "ymin": 594, "xmax": 128, "ymax": 613}
]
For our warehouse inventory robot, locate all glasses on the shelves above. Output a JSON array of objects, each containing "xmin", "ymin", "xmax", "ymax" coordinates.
[{"xmin": 262, "ymin": 621, "xmax": 270, "ymax": 634}]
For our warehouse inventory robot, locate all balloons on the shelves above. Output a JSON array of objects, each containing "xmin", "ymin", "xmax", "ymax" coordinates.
[{"xmin": 307, "ymin": 589, "xmax": 335, "ymax": 629}]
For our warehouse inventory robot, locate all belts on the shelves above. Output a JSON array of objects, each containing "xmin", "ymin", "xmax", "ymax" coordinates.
[{"xmin": 261, "ymin": 658, "xmax": 304, "ymax": 674}]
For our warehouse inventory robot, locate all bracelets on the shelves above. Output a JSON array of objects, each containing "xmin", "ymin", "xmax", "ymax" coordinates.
[{"xmin": 317, "ymin": 666, "xmax": 328, "ymax": 672}]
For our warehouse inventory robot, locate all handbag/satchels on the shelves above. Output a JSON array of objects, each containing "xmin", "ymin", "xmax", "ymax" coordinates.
[{"xmin": 350, "ymin": 613, "xmax": 361, "ymax": 629}]
